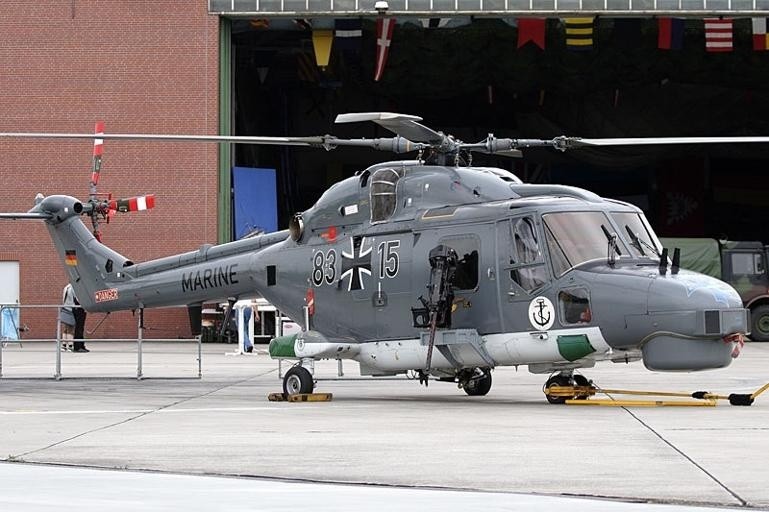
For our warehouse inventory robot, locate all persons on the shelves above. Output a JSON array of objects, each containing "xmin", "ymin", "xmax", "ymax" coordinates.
[
  {"xmin": 225, "ymin": 299, "xmax": 260, "ymax": 352},
  {"xmin": 58, "ymin": 283, "xmax": 74, "ymax": 352},
  {"xmin": 71, "ymin": 290, "xmax": 90, "ymax": 352}
]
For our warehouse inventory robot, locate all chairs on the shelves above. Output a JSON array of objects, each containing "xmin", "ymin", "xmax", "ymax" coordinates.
[
  {"xmin": 61, "ymin": 345, "xmax": 89, "ymax": 352},
  {"xmin": 247, "ymin": 346, "xmax": 253, "ymax": 353}
]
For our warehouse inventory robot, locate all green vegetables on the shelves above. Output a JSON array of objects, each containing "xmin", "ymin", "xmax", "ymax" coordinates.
[{"xmin": 0, "ymin": 111, "xmax": 768, "ymax": 404}]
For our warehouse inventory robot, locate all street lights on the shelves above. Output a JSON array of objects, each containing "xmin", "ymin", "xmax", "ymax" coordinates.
[{"xmin": 60, "ymin": 307, "xmax": 76, "ymax": 327}]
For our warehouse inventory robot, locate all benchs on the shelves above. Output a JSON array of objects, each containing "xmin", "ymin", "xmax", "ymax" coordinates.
[
  {"xmin": 741, "ymin": 16, "xmax": 769, "ymax": 51},
  {"xmin": 657, "ymin": 17, "xmax": 684, "ymax": 51},
  {"xmin": 563, "ymin": 18, "xmax": 594, "ymax": 52},
  {"xmin": 334, "ymin": 19, "xmax": 363, "ymax": 49},
  {"xmin": 704, "ymin": 18, "xmax": 735, "ymax": 52}
]
